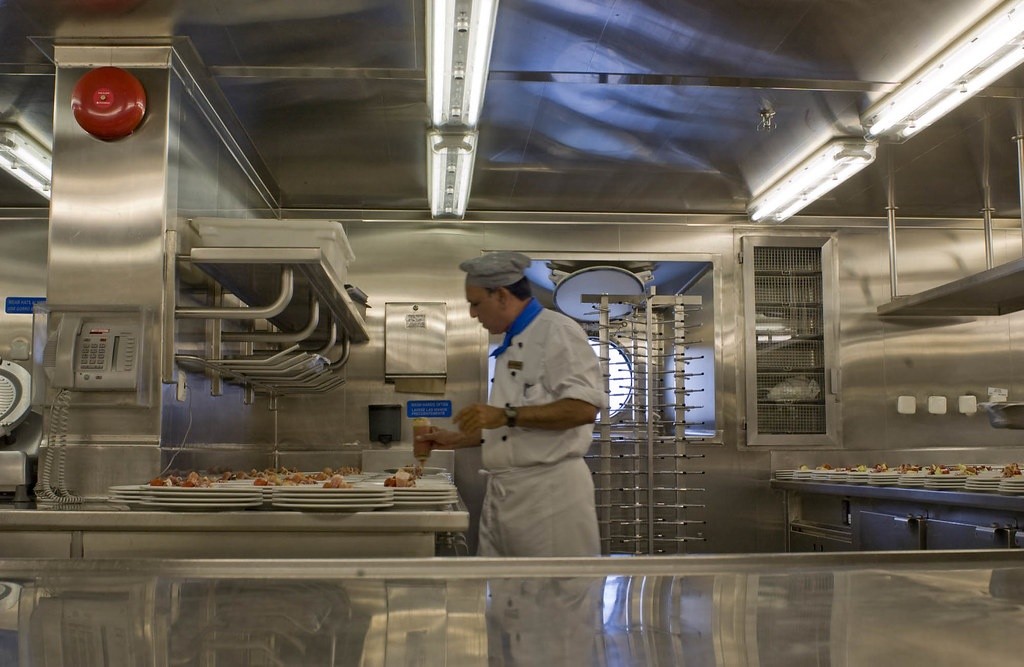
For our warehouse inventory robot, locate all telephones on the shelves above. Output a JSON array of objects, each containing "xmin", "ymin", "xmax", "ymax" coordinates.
[{"xmin": 45, "ymin": 310, "xmax": 141, "ymax": 390}]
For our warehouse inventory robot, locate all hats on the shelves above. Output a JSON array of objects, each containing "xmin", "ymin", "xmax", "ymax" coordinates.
[{"xmin": 459, "ymin": 251, "xmax": 531, "ymax": 288}]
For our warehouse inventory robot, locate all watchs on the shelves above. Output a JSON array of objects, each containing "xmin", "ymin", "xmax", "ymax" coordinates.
[{"xmin": 504, "ymin": 403, "xmax": 518, "ymax": 427}]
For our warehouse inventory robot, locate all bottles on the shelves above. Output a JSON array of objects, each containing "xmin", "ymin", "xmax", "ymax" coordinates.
[{"xmin": 412, "ymin": 417, "xmax": 433, "ymax": 468}]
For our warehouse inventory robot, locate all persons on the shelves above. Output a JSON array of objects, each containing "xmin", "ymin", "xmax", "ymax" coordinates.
[
  {"xmin": 415, "ymin": 252, "xmax": 608, "ymax": 558},
  {"xmin": 487, "ymin": 576, "xmax": 606, "ymax": 667}
]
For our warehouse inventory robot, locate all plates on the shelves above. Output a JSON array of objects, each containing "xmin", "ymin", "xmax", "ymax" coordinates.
[
  {"xmin": 107, "ymin": 468, "xmax": 459, "ymax": 511},
  {"xmin": 774, "ymin": 467, "xmax": 1024, "ymax": 495}
]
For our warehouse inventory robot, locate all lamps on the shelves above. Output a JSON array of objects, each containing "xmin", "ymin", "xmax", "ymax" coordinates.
[
  {"xmin": 860, "ymin": 0, "xmax": 1024, "ymax": 145},
  {"xmin": 0, "ymin": 123, "xmax": 52, "ymax": 200},
  {"xmin": 427, "ymin": 129, "xmax": 480, "ymax": 220},
  {"xmin": 745, "ymin": 137, "xmax": 879, "ymax": 224},
  {"xmin": 425, "ymin": 0, "xmax": 499, "ymax": 132}
]
